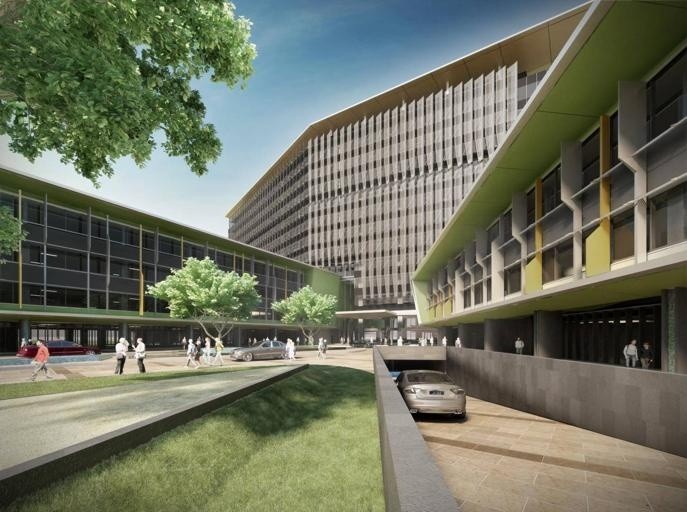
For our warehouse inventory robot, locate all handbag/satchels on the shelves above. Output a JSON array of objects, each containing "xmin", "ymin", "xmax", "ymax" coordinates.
[
  {"xmin": 136, "ymin": 352, "xmax": 146, "ymax": 358},
  {"xmin": 116, "ymin": 353, "xmax": 124, "ymax": 360}
]
